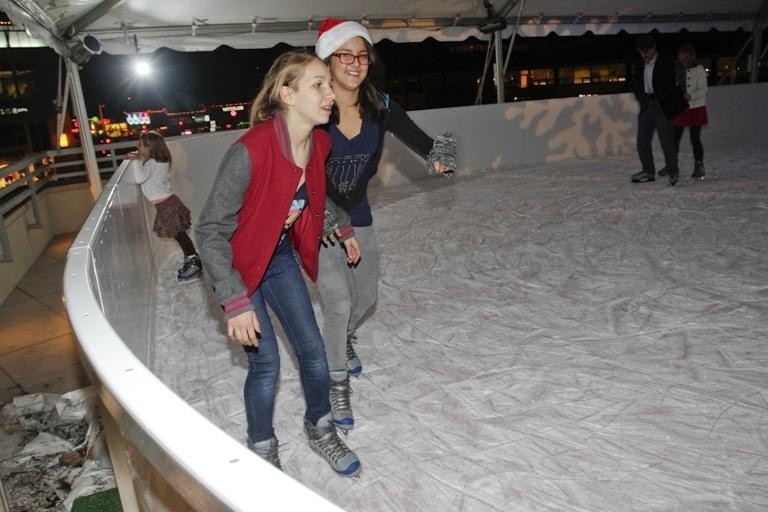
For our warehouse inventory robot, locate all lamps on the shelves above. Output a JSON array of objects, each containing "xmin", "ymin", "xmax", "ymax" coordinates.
[{"xmin": 65, "ymin": 32, "xmax": 103, "ymax": 67}]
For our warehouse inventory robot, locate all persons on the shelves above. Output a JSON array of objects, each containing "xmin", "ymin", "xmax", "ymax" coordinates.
[
  {"xmin": 194, "ymin": 54, "xmax": 362, "ymax": 480},
  {"xmin": 123, "ymin": 128, "xmax": 202, "ymax": 280},
  {"xmin": 295, "ymin": 14, "xmax": 458, "ymax": 430},
  {"xmin": 658, "ymin": 46, "xmax": 710, "ymax": 177},
  {"xmin": 629, "ymin": 35, "xmax": 689, "ymax": 185}
]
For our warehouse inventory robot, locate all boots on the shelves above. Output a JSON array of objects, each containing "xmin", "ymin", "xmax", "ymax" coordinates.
[
  {"xmin": 657, "ymin": 167, "xmax": 671, "ymax": 176},
  {"xmin": 692, "ymin": 161, "xmax": 706, "ymax": 177}
]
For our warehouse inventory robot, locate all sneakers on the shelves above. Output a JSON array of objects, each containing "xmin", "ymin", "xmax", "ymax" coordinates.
[
  {"xmin": 246, "ymin": 434, "xmax": 281, "ymax": 469},
  {"xmin": 631, "ymin": 170, "xmax": 655, "ymax": 181},
  {"xmin": 303, "ymin": 411, "xmax": 361, "ymax": 477},
  {"xmin": 346, "ymin": 335, "xmax": 362, "ymax": 376},
  {"xmin": 177, "ymin": 261, "xmax": 188, "ymax": 274},
  {"xmin": 328, "ymin": 369, "xmax": 354, "ymax": 429},
  {"xmin": 668, "ymin": 175, "xmax": 678, "ymax": 184},
  {"xmin": 177, "ymin": 253, "xmax": 202, "ymax": 281}
]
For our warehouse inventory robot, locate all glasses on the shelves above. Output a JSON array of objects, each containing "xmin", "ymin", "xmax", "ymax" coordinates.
[{"xmin": 333, "ymin": 52, "xmax": 372, "ymax": 66}]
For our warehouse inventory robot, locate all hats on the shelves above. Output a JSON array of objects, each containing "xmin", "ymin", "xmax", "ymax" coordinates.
[{"xmin": 314, "ymin": 18, "xmax": 373, "ymax": 60}]
